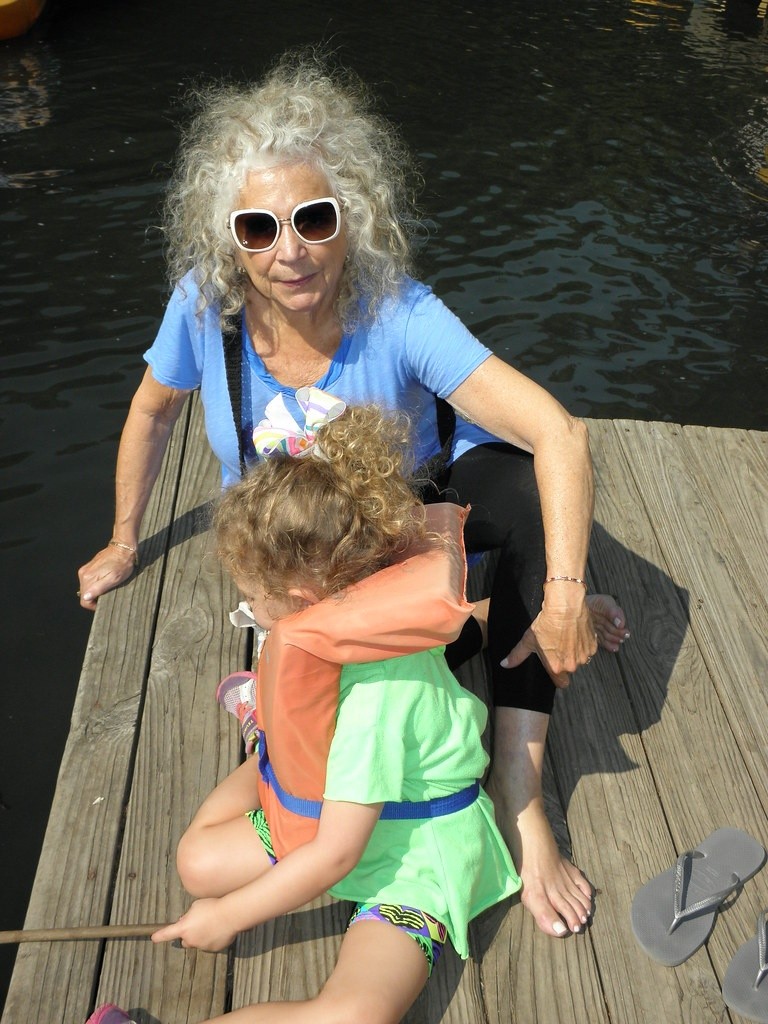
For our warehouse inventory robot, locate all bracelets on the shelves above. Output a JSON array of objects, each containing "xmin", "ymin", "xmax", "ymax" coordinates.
[
  {"xmin": 542, "ymin": 575, "xmax": 591, "ymax": 590},
  {"xmin": 108, "ymin": 540, "xmax": 140, "ymax": 567}
]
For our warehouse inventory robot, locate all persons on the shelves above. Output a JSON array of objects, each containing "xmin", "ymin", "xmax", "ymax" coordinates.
[
  {"xmin": 87, "ymin": 403, "xmax": 523, "ymax": 1024},
  {"xmin": 75, "ymin": 63, "xmax": 632, "ymax": 938}
]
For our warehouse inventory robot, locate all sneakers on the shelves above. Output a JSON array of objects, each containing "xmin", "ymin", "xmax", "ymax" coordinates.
[{"xmin": 214, "ymin": 670, "xmax": 262, "ymax": 758}]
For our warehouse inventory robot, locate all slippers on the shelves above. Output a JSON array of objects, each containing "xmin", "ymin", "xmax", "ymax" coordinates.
[
  {"xmin": 722, "ymin": 910, "xmax": 768, "ymax": 1019},
  {"xmin": 629, "ymin": 827, "xmax": 766, "ymax": 968}
]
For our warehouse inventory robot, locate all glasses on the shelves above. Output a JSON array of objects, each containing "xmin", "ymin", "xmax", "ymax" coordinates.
[{"xmin": 226, "ymin": 197, "xmax": 346, "ymax": 253}]
